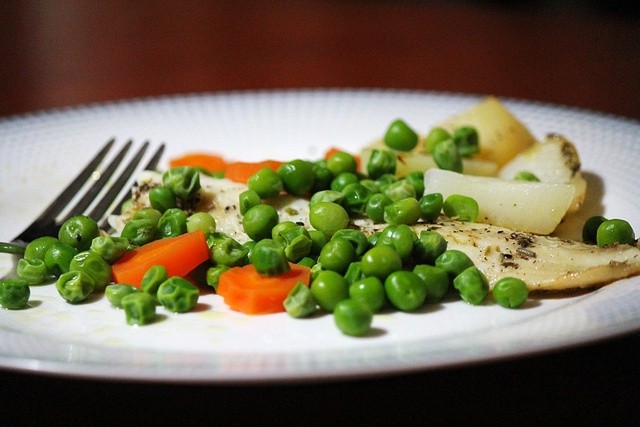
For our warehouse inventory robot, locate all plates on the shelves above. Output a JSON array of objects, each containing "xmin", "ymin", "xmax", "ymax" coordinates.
[{"xmin": 0, "ymin": 86, "xmax": 640, "ymax": 386}]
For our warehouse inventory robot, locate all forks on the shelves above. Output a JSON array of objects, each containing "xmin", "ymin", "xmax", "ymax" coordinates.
[{"xmin": 0, "ymin": 136, "xmax": 165, "ymax": 295}]
